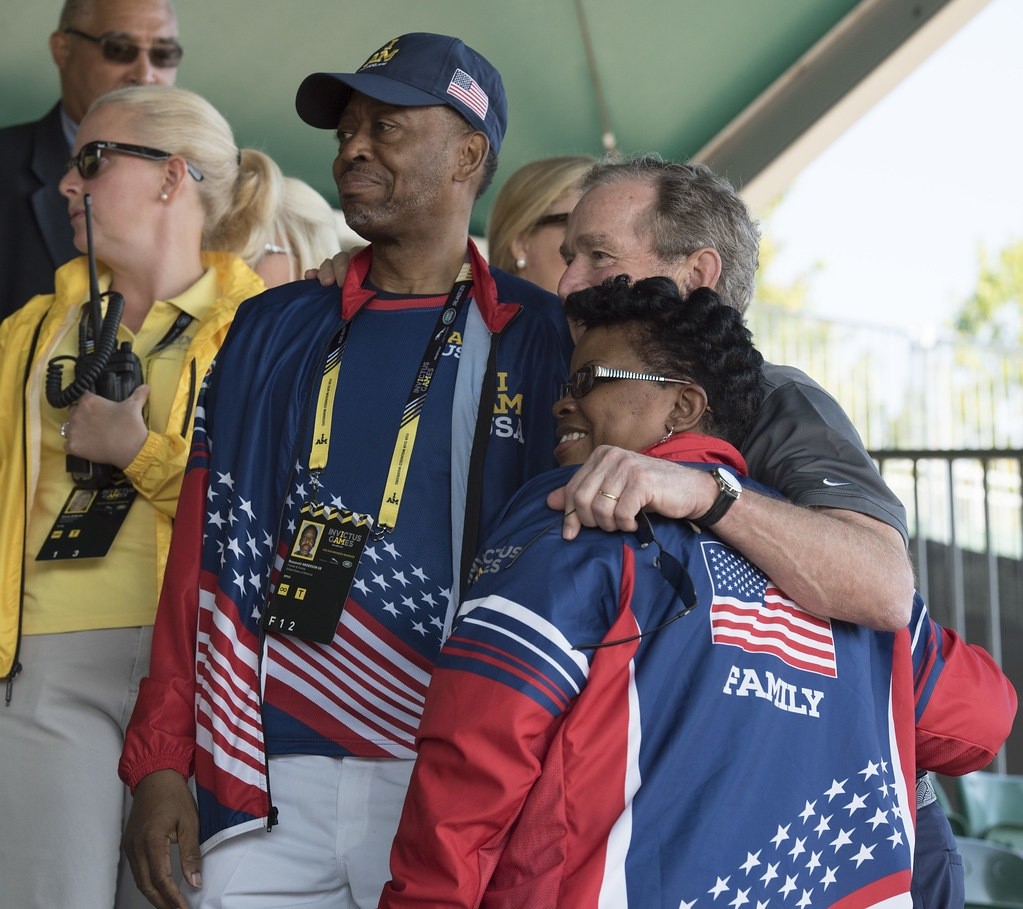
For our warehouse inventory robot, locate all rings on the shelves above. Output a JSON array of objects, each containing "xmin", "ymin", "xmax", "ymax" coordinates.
[
  {"xmin": 61, "ymin": 422, "xmax": 70, "ymax": 437},
  {"xmin": 598, "ymin": 491, "xmax": 620, "ymax": 501}
]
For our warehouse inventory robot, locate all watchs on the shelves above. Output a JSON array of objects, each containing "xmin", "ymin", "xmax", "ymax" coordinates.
[{"xmin": 687, "ymin": 468, "xmax": 742, "ymax": 533}]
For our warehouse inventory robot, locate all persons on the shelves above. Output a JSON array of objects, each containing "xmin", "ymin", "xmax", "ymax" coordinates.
[
  {"xmin": 1, "ymin": 0, "xmax": 182, "ymax": 323},
  {"xmin": 378, "ymin": 273, "xmax": 1018, "ymax": 908},
  {"xmin": 0, "ymin": 86, "xmax": 283, "ymax": 909},
  {"xmin": 491, "ymin": 155, "xmax": 596, "ymax": 297},
  {"xmin": 306, "ymin": 159, "xmax": 965, "ymax": 908},
  {"xmin": 249, "ymin": 178, "xmax": 360, "ymax": 287},
  {"xmin": 295, "ymin": 525, "xmax": 317, "ymax": 557},
  {"xmin": 118, "ymin": 32, "xmax": 573, "ymax": 909}
]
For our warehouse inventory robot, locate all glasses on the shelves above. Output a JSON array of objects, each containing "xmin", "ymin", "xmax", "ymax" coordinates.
[
  {"xmin": 61, "ymin": 140, "xmax": 204, "ymax": 182},
  {"xmin": 63, "ymin": 27, "xmax": 184, "ymax": 68},
  {"xmin": 559, "ymin": 364, "xmax": 714, "ymax": 411}
]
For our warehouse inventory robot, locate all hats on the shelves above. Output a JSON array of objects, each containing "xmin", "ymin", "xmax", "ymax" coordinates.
[{"xmin": 296, "ymin": 32, "xmax": 507, "ymax": 158}]
